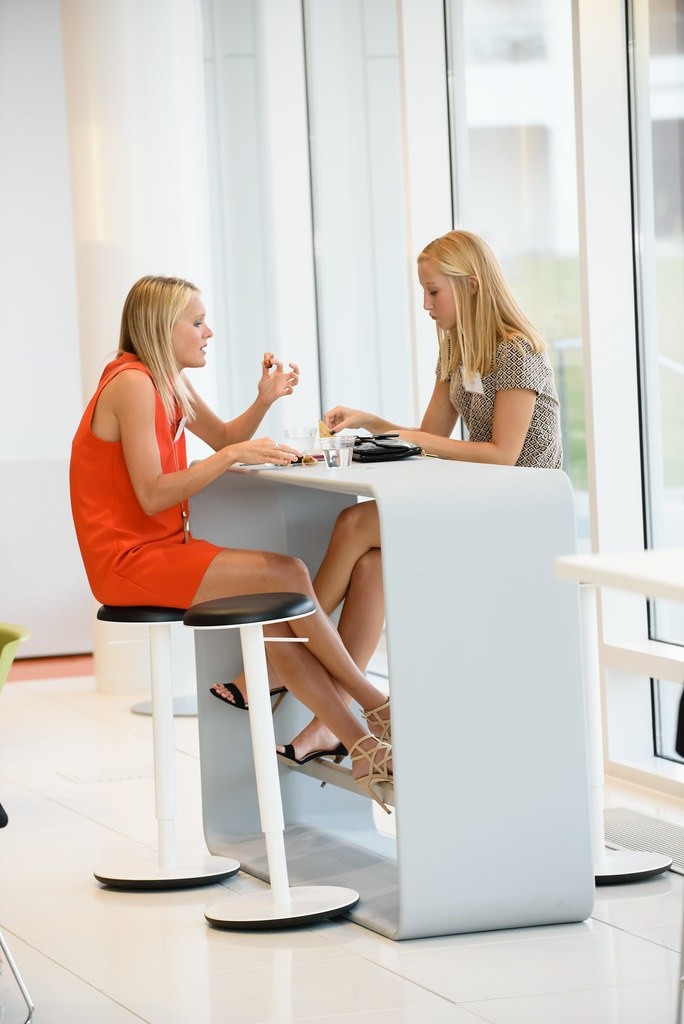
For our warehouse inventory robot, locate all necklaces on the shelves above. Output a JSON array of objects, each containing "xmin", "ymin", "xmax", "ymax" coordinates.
[{"xmin": 170, "ymin": 423, "xmax": 189, "ymax": 545}]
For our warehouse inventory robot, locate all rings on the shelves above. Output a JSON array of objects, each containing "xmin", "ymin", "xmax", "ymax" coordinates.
[{"xmin": 275, "ymin": 442, "xmax": 280, "ymax": 449}]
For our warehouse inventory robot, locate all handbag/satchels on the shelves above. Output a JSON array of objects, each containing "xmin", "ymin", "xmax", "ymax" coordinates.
[{"xmin": 353, "ymin": 434, "xmax": 423, "ymax": 463}]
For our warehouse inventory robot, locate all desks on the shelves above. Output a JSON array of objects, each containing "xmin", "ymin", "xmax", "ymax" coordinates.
[{"xmin": 191, "ymin": 449, "xmax": 594, "ymax": 943}]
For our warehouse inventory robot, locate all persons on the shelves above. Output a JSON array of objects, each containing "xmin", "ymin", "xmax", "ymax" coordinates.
[
  {"xmin": 69, "ymin": 276, "xmax": 394, "ymax": 815},
  {"xmin": 209, "ymin": 231, "xmax": 563, "ymax": 788}
]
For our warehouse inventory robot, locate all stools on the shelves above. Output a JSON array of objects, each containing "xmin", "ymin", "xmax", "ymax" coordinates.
[
  {"xmin": 183, "ymin": 590, "xmax": 360, "ymax": 932},
  {"xmin": 91, "ymin": 602, "xmax": 241, "ymax": 889}
]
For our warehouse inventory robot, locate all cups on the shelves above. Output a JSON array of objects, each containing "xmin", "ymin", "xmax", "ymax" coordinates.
[
  {"xmin": 319, "ymin": 436, "xmax": 357, "ymax": 469},
  {"xmin": 282, "ymin": 427, "xmax": 318, "ymax": 452}
]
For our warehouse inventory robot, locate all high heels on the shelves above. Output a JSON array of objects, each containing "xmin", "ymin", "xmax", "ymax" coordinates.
[
  {"xmin": 210, "ymin": 683, "xmax": 288, "ymax": 716},
  {"xmin": 275, "ymin": 741, "xmax": 349, "ymax": 788},
  {"xmin": 349, "ymin": 735, "xmax": 394, "ymax": 815},
  {"xmin": 360, "ymin": 696, "xmax": 391, "ymax": 744}
]
